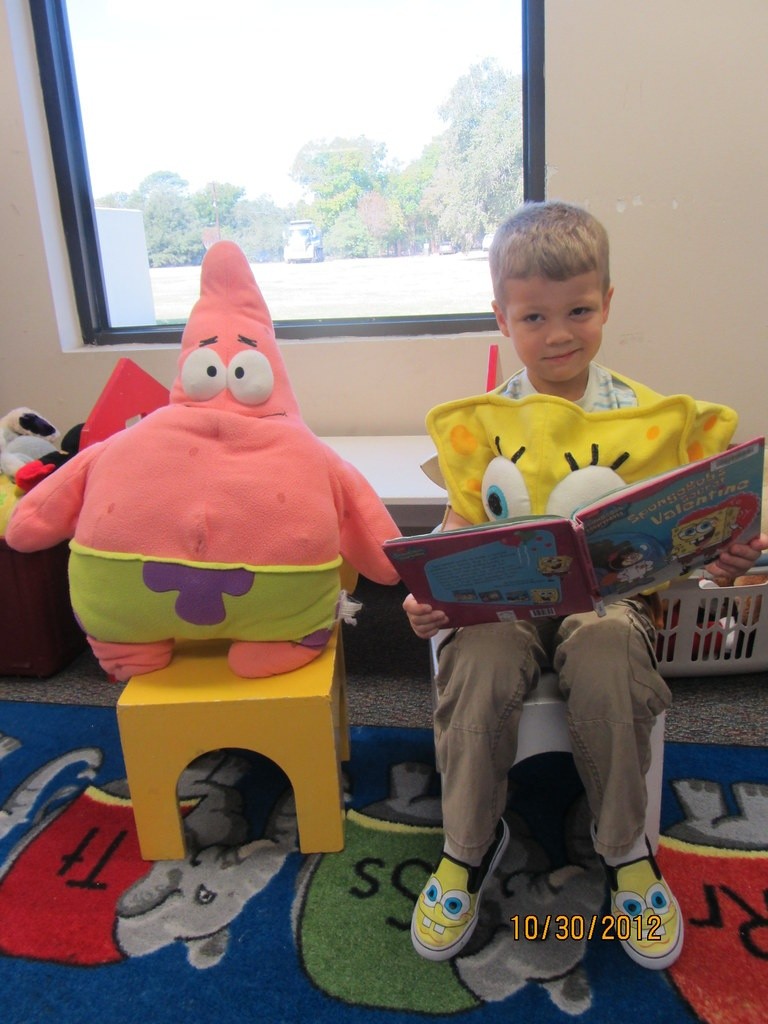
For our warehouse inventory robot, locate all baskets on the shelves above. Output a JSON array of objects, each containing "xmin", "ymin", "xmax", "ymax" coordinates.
[{"xmin": 628, "ymin": 578, "xmax": 768, "ymax": 677}]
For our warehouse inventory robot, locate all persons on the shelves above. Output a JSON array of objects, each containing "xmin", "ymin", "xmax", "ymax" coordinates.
[{"xmin": 402, "ymin": 203, "xmax": 768, "ymax": 972}]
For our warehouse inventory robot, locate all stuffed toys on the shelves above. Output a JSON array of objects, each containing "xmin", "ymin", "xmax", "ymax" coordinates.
[
  {"xmin": 0, "ymin": 406, "xmax": 84, "ymax": 541},
  {"xmin": 6, "ymin": 241, "xmax": 402, "ymax": 679},
  {"xmin": 419, "ymin": 398, "xmax": 740, "ymax": 592},
  {"xmin": 658, "ymin": 575, "xmax": 767, "ymax": 656}
]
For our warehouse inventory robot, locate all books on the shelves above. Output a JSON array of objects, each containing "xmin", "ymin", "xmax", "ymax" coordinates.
[{"xmin": 384, "ymin": 435, "xmax": 765, "ymax": 629}]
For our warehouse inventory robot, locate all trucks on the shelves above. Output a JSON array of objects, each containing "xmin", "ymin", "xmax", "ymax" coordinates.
[{"xmin": 281, "ymin": 219, "xmax": 325, "ymax": 263}]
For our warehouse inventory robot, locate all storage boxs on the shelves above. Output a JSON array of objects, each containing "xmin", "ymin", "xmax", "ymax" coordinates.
[
  {"xmin": 649, "ymin": 572, "xmax": 767, "ymax": 675},
  {"xmin": 0, "ymin": 528, "xmax": 84, "ymax": 680}
]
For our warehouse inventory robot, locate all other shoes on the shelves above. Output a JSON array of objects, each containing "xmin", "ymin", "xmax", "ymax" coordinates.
[
  {"xmin": 594, "ymin": 835, "xmax": 683, "ymax": 969},
  {"xmin": 408, "ymin": 816, "xmax": 508, "ymax": 961}
]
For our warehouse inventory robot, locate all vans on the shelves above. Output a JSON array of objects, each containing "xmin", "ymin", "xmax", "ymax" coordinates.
[{"xmin": 482, "ymin": 234, "xmax": 494, "ymax": 252}]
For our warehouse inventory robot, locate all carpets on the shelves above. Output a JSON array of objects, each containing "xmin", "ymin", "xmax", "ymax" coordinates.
[{"xmin": 0, "ymin": 696, "xmax": 768, "ymax": 1024}]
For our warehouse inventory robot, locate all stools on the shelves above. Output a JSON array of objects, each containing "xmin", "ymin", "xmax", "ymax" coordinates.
[
  {"xmin": 423, "ymin": 523, "xmax": 671, "ymax": 855},
  {"xmin": 116, "ymin": 604, "xmax": 353, "ymax": 860}
]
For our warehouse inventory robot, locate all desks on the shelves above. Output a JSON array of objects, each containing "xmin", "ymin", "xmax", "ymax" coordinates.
[{"xmin": 308, "ymin": 432, "xmax": 453, "ymax": 529}]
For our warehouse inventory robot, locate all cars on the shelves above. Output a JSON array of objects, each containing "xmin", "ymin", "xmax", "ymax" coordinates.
[{"xmin": 439, "ymin": 242, "xmax": 457, "ymax": 255}]
